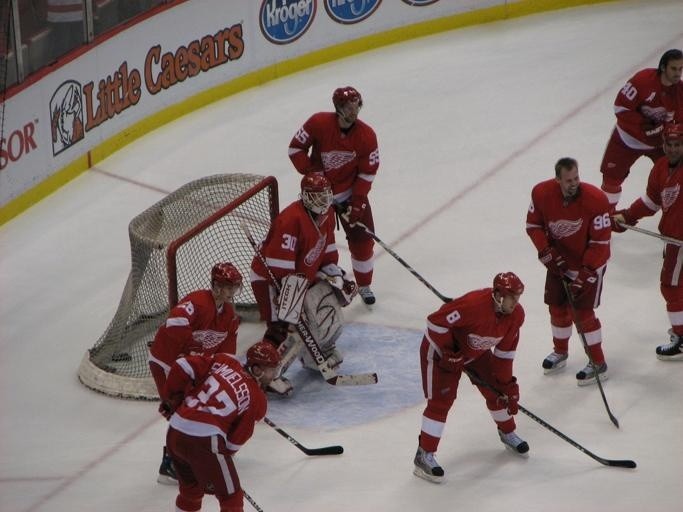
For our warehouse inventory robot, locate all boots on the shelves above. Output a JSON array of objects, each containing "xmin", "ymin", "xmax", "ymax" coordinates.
[
  {"xmin": 654, "ymin": 327, "xmax": 683, "ymax": 356},
  {"xmin": 412, "ymin": 444, "xmax": 445, "ymax": 477},
  {"xmin": 157, "ymin": 445, "xmax": 179, "ymax": 481},
  {"xmin": 495, "ymin": 426, "xmax": 530, "ymax": 454}
]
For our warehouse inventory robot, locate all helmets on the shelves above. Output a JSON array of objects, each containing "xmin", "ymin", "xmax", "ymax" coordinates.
[
  {"xmin": 244, "ymin": 337, "xmax": 280, "ymax": 370},
  {"xmin": 209, "ymin": 260, "xmax": 242, "ymax": 287},
  {"xmin": 661, "ymin": 122, "xmax": 683, "ymax": 141},
  {"xmin": 331, "ymin": 86, "xmax": 362, "ymax": 109},
  {"xmin": 492, "ymin": 272, "xmax": 523, "ymax": 297},
  {"xmin": 300, "ymin": 173, "xmax": 332, "ymax": 195}
]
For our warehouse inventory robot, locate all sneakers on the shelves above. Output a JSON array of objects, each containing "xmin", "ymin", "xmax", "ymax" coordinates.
[
  {"xmin": 575, "ymin": 362, "xmax": 607, "ymax": 381},
  {"xmin": 540, "ymin": 351, "xmax": 569, "ymax": 370},
  {"xmin": 357, "ymin": 285, "xmax": 376, "ymax": 305}
]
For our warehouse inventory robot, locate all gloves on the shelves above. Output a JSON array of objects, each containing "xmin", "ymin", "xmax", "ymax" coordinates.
[
  {"xmin": 495, "ymin": 375, "xmax": 520, "ymax": 416},
  {"xmin": 538, "ymin": 246, "xmax": 569, "ymax": 276},
  {"xmin": 609, "ymin": 209, "xmax": 637, "ymax": 233},
  {"xmin": 344, "ymin": 197, "xmax": 368, "ymax": 228},
  {"xmin": 437, "ymin": 345, "xmax": 465, "ymax": 381},
  {"xmin": 158, "ymin": 398, "xmax": 180, "ymax": 421},
  {"xmin": 568, "ymin": 266, "xmax": 599, "ymax": 303}
]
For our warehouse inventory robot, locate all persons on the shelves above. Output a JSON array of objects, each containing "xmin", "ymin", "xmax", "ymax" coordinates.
[
  {"xmin": 612, "ymin": 121, "xmax": 683, "ymax": 357},
  {"xmin": 287, "ymin": 86, "xmax": 380, "ymax": 302},
  {"xmin": 244, "ymin": 172, "xmax": 359, "ymax": 399},
  {"xmin": 598, "ymin": 48, "xmax": 682, "ymax": 213},
  {"xmin": 413, "ymin": 272, "xmax": 538, "ymax": 478},
  {"xmin": 521, "ymin": 159, "xmax": 609, "ymax": 380},
  {"xmin": 158, "ymin": 343, "xmax": 280, "ymax": 512},
  {"xmin": 143, "ymin": 263, "xmax": 240, "ymax": 481}
]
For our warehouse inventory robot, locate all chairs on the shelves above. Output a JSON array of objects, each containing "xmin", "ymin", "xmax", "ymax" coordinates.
[{"xmin": 0, "ymin": 0, "xmax": 117, "ymax": 94}]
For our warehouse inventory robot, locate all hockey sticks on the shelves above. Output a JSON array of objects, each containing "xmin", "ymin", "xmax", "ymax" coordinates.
[
  {"xmin": 263, "ymin": 417, "xmax": 343, "ymax": 456},
  {"xmin": 560, "ymin": 278, "xmax": 620, "ymax": 430},
  {"xmin": 239, "ymin": 223, "xmax": 378, "ymax": 387},
  {"xmin": 329, "ymin": 200, "xmax": 454, "ymax": 302},
  {"xmin": 463, "ymin": 365, "xmax": 636, "ymax": 468}
]
